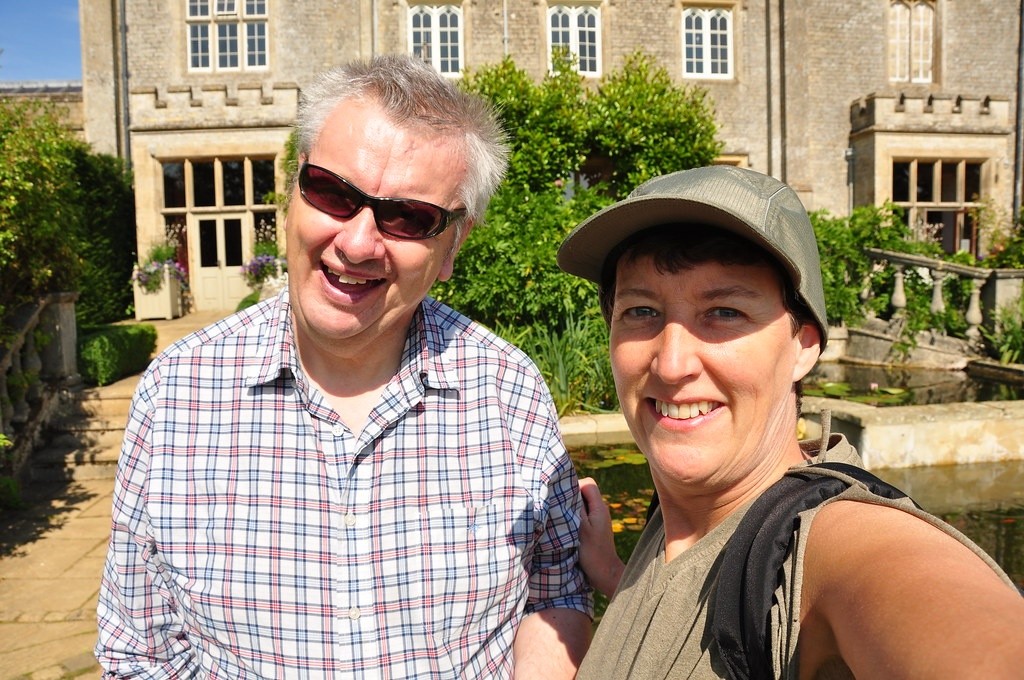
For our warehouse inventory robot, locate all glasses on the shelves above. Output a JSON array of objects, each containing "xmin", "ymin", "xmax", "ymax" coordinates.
[{"xmin": 298, "ymin": 155, "xmax": 467, "ymax": 242}]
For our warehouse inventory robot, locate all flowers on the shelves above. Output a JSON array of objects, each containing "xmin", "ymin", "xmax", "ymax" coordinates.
[
  {"xmin": 128, "ymin": 260, "xmax": 190, "ymax": 295},
  {"xmin": 242, "ymin": 253, "xmax": 288, "ymax": 283}
]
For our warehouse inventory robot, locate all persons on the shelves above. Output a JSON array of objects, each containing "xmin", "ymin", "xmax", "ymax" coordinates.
[
  {"xmin": 96, "ymin": 52, "xmax": 593, "ymax": 680},
  {"xmin": 557, "ymin": 163, "xmax": 1024, "ymax": 680}
]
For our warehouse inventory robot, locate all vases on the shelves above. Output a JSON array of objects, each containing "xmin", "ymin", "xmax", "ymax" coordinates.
[{"xmin": 130, "ymin": 264, "xmax": 183, "ymax": 321}]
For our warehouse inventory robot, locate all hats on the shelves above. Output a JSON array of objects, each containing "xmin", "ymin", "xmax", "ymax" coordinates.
[{"xmin": 556, "ymin": 165, "xmax": 829, "ymax": 356}]
